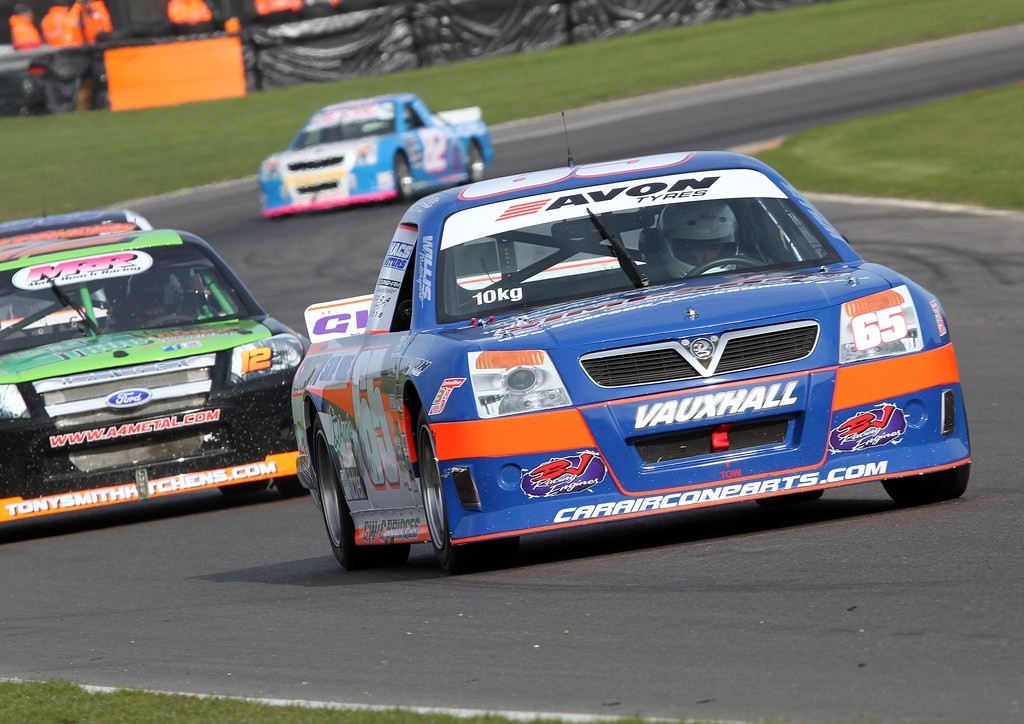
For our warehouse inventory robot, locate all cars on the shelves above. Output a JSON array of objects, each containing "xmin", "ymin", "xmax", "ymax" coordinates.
[
  {"xmin": 291, "ymin": 149, "xmax": 973, "ymax": 574},
  {"xmin": 0, "ymin": 208, "xmax": 155, "ymax": 331},
  {"xmin": 0, "ymin": 229, "xmax": 310, "ymax": 528},
  {"xmin": 259, "ymin": 93, "xmax": 493, "ymax": 218}
]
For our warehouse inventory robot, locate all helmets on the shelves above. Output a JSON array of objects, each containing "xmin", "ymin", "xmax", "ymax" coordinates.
[
  {"xmin": 657, "ymin": 199, "xmax": 739, "ymax": 278},
  {"xmin": 127, "ymin": 271, "xmax": 184, "ymax": 328}
]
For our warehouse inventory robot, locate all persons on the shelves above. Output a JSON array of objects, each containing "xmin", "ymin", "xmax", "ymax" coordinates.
[
  {"xmin": 636, "ymin": 200, "xmax": 740, "ymax": 285},
  {"xmin": 117, "ymin": 269, "xmax": 193, "ymax": 330}
]
